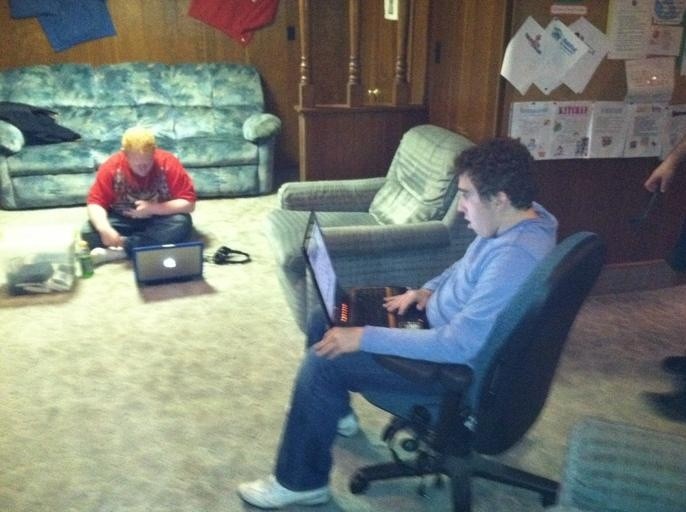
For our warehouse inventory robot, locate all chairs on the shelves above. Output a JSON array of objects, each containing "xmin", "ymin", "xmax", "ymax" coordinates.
[{"xmin": 349, "ymin": 231, "xmax": 605, "ymax": 512}]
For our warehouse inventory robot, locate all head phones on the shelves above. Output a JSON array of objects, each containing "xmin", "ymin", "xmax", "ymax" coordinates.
[{"xmin": 212, "ymin": 246, "xmax": 250, "ymax": 264}]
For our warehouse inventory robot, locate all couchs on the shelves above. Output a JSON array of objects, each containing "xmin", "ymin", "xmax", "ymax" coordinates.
[
  {"xmin": 263, "ymin": 123, "xmax": 479, "ymax": 336},
  {"xmin": 0, "ymin": 60, "xmax": 281, "ymax": 212}
]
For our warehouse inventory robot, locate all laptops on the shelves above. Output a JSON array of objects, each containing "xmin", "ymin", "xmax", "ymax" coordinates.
[
  {"xmin": 131, "ymin": 241, "xmax": 204, "ymax": 286},
  {"xmin": 301, "ymin": 210, "xmax": 427, "ymax": 331}
]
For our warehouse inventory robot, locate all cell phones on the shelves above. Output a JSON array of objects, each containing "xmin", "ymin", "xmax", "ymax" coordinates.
[{"xmin": 110, "ymin": 202, "xmax": 136, "ymax": 214}]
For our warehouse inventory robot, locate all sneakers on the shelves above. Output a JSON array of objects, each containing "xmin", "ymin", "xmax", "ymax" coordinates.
[
  {"xmin": 283, "ymin": 401, "xmax": 360, "ymax": 438},
  {"xmin": 236, "ymin": 473, "xmax": 331, "ymax": 510},
  {"xmin": 641, "ymin": 390, "xmax": 685, "ymax": 422},
  {"xmin": 660, "ymin": 356, "xmax": 686, "ymax": 375}
]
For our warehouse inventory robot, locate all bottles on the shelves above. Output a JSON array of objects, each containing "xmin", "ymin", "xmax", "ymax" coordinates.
[{"xmin": 74, "ymin": 241, "xmax": 95, "ymax": 278}]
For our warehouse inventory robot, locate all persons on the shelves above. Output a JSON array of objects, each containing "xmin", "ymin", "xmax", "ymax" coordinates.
[
  {"xmin": 646, "ymin": 135, "xmax": 686, "ymax": 420},
  {"xmin": 79, "ymin": 127, "xmax": 197, "ymax": 265},
  {"xmin": 236, "ymin": 140, "xmax": 558, "ymax": 509}
]
